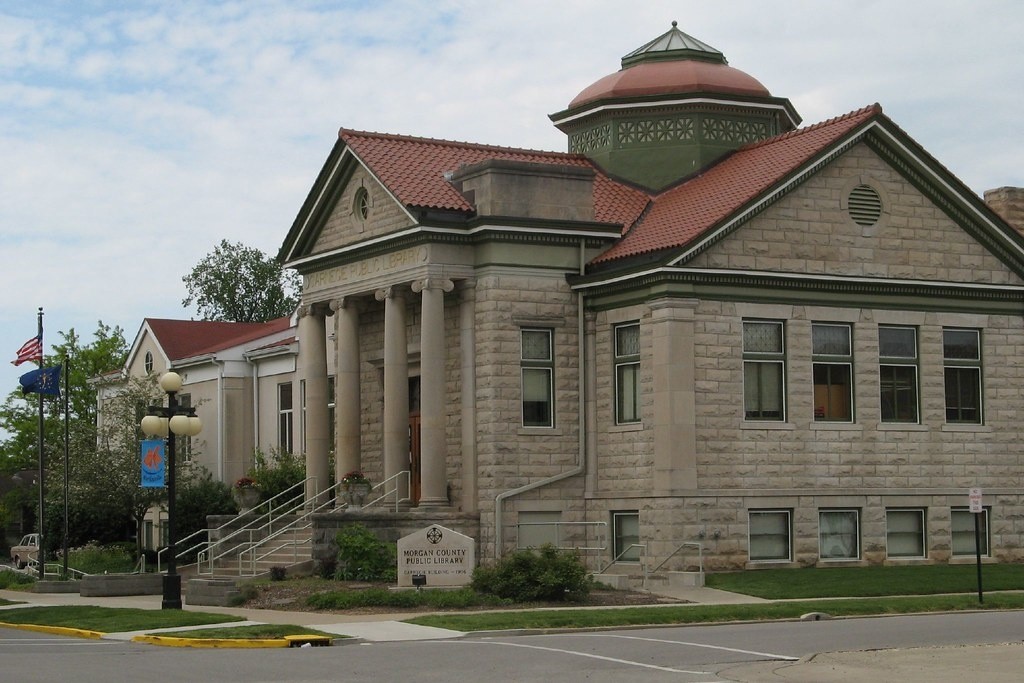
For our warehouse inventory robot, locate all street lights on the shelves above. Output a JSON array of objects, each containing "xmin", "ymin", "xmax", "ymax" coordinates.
[{"xmin": 141, "ymin": 369, "xmax": 203, "ymax": 613}]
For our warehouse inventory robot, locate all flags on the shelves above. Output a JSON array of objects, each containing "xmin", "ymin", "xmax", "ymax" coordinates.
[
  {"xmin": 11, "ymin": 327, "xmax": 43, "ymax": 366},
  {"xmin": 19, "ymin": 364, "xmax": 63, "ymax": 397}
]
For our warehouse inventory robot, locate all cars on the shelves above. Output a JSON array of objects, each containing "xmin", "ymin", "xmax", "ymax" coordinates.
[{"xmin": 10, "ymin": 533, "xmax": 43, "ymax": 570}]
